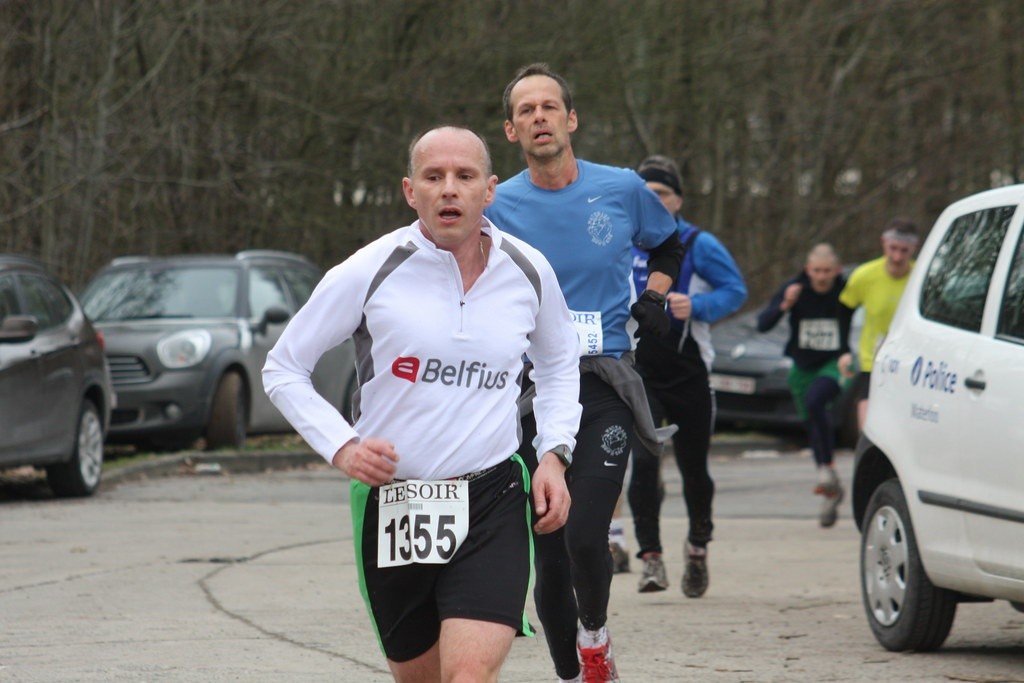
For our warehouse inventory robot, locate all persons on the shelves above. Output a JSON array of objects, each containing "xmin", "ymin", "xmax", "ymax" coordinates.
[
  {"xmin": 481, "ymin": 69, "xmax": 683, "ymax": 683},
  {"xmin": 602, "ymin": 151, "xmax": 749, "ymax": 598},
  {"xmin": 836, "ymin": 221, "xmax": 924, "ymax": 427},
  {"xmin": 760, "ymin": 243, "xmax": 861, "ymax": 527},
  {"xmin": 261, "ymin": 122, "xmax": 582, "ymax": 683}
]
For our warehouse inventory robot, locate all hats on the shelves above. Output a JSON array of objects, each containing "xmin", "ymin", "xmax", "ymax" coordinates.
[{"xmin": 637, "ymin": 155, "xmax": 682, "ymax": 197}]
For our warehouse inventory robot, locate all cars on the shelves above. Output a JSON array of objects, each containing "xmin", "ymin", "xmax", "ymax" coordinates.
[
  {"xmin": 79, "ymin": 247, "xmax": 361, "ymax": 452},
  {"xmin": 846, "ymin": 182, "xmax": 1023, "ymax": 657},
  {"xmin": 706, "ymin": 264, "xmax": 872, "ymax": 446},
  {"xmin": 0, "ymin": 250, "xmax": 119, "ymax": 501}
]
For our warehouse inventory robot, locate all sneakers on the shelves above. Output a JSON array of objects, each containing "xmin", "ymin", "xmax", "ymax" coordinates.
[
  {"xmin": 638, "ymin": 555, "xmax": 669, "ymax": 592},
  {"xmin": 556, "ymin": 663, "xmax": 584, "ymax": 683},
  {"xmin": 812, "ymin": 465, "xmax": 840, "ymax": 498},
  {"xmin": 820, "ymin": 487, "xmax": 843, "ymax": 527},
  {"xmin": 576, "ymin": 624, "xmax": 620, "ymax": 683},
  {"xmin": 681, "ymin": 536, "xmax": 708, "ymax": 598}
]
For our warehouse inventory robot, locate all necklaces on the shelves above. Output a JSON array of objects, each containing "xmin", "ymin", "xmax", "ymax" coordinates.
[{"xmin": 478, "ymin": 239, "xmax": 485, "ymax": 277}]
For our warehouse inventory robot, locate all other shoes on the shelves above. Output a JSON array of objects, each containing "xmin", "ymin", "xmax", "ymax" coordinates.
[{"xmin": 608, "ymin": 541, "xmax": 629, "ymax": 573}]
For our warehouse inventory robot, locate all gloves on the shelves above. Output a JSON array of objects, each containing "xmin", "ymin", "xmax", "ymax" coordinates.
[{"xmin": 631, "ymin": 290, "xmax": 674, "ymax": 353}]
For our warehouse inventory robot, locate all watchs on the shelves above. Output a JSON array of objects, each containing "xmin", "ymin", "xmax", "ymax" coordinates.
[{"xmin": 548, "ymin": 444, "xmax": 573, "ymax": 467}]
